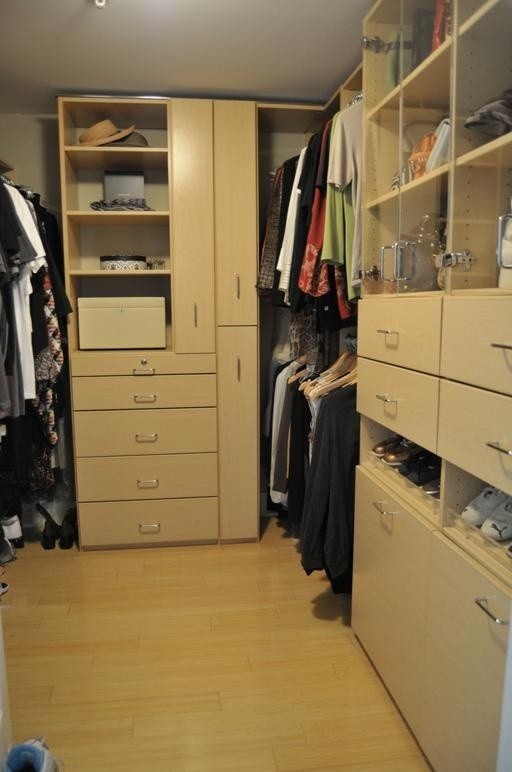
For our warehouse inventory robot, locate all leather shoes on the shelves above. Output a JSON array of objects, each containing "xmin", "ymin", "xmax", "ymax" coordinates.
[{"xmin": 1, "ymin": 512, "xmax": 24, "ymax": 596}]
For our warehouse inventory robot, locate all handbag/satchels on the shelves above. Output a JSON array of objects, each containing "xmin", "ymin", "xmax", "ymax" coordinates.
[{"xmin": 391, "ymin": 117, "xmax": 451, "ymax": 189}]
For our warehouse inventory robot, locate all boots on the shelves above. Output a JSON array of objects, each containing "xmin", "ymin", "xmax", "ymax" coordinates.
[
  {"xmin": 59, "ymin": 508, "xmax": 76, "ymax": 548},
  {"xmin": 35, "ymin": 502, "xmax": 62, "ymax": 549}
]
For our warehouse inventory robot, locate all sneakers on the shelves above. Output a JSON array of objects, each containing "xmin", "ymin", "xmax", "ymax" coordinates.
[
  {"xmin": 461, "ymin": 486, "xmax": 510, "ymax": 526},
  {"xmin": 406, "ymin": 459, "xmax": 441, "ymax": 488},
  {"xmin": 381, "ymin": 438, "xmax": 424, "ymax": 466},
  {"xmin": 395, "ymin": 450, "xmax": 435, "ymax": 476},
  {"xmin": 370, "ymin": 433, "xmax": 404, "ymax": 457},
  {"xmin": 421, "ymin": 478, "xmax": 440, "ymax": 494},
  {"xmin": 480, "ymin": 496, "xmax": 512, "ymax": 541}
]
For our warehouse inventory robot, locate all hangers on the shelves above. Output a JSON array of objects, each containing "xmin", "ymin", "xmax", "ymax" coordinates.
[
  {"xmin": 0, "ymin": 173, "xmax": 27, "ymax": 191},
  {"xmin": 287, "ymin": 336, "xmax": 358, "ymax": 400},
  {"xmin": 344, "ymin": 91, "xmax": 364, "ymax": 108}
]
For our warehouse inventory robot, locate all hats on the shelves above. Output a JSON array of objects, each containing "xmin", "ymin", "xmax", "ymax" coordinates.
[
  {"xmin": 104, "ymin": 131, "xmax": 148, "ymax": 147},
  {"xmin": 69, "ymin": 118, "xmax": 135, "ymax": 146}
]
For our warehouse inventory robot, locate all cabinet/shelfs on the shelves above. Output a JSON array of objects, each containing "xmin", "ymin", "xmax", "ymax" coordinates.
[
  {"xmin": 58, "ymin": 93, "xmax": 262, "ymax": 545},
  {"xmin": 350, "ymin": 0, "xmax": 512, "ymax": 772}
]
